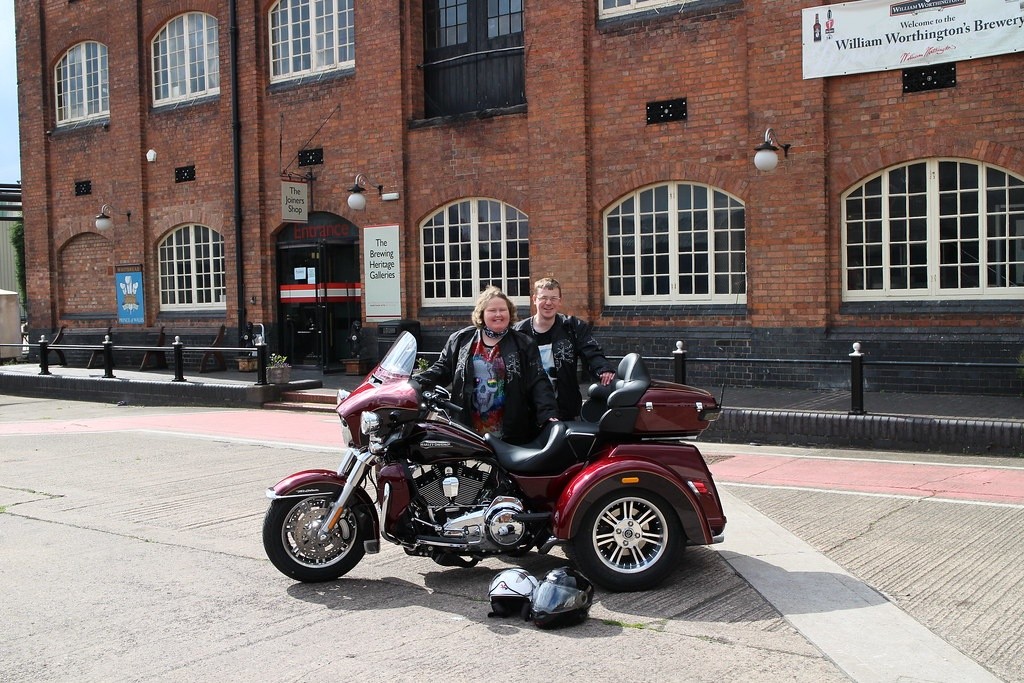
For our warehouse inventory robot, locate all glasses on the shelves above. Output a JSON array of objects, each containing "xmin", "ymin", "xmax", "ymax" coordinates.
[{"xmin": 536, "ymin": 297, "xmax": 560, "ymax": 303}]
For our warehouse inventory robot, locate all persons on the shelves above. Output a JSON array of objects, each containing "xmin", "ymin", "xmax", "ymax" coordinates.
[
  {"xmin": 511, "ymin": 278, "xmax": 616, "ymax": 418},
  {"xmin": 413, "ymin": 286, "xmax": 560, "ymax": 446}
]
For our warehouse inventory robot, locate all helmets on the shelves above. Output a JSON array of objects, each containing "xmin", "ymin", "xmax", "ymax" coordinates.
[
  {"xmin": 531, "ymin": 566, "xmax": 594, "ymax": 629},
  {"xmin": 488, "ymin": 568, "xmax": 538, "ymax": 622}
]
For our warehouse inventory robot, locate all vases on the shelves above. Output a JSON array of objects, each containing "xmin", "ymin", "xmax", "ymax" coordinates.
[{"xmin": 266, "ymin": 365, "xmax": 292, "ymax": 384}]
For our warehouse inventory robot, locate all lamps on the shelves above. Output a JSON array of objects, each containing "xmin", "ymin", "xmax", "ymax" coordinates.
[
  {"xmin": 94, "ymin": 204, "xmax": 131, "ymax": 230},
  {"xmin": 754, "ymin": 127, "xmax": 791, "ymax": 172},
  {"xmin": 346, "ymin": 173, "xmax": 383, "ymax": 210}
]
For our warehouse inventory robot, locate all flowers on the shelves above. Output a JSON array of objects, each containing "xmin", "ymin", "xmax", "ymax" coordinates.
[{"xmin": 266, "ymin": 353, "xmax": 289, "ymax": 367}]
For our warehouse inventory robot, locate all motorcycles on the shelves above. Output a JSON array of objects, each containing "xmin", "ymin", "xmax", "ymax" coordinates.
[{"xmin": 262, "ymin": 331, "xmax": 726, "ymax": 594}]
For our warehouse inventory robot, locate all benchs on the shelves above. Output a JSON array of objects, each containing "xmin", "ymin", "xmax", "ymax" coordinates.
[{"xmin": 39, "ymin": 325, "xmax": 228, "ymax": 373}]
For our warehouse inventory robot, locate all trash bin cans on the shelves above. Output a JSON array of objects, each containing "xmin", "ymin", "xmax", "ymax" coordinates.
[{"xmin": 376, "ymin": 319, "xmax": 422, "ymax": 364}]
